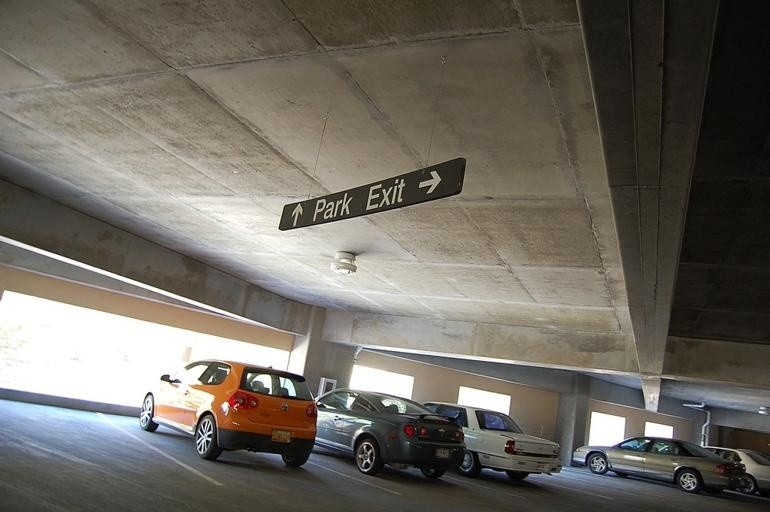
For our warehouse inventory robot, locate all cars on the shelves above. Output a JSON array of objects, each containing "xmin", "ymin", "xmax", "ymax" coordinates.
[
  {"xmin": 420, "ymin": 400, "xmax": 562, "ymax": 480},
  {"xmin": 702, "ymin": 444, "xmax": 770, "ymax": 494},
  {"xmin": 571, "ymin": 436, "xmax": 745, "ymax": 494},
  {"xmin": 311, "ymin": 387, "xmax": 465, "ymax": 480}
]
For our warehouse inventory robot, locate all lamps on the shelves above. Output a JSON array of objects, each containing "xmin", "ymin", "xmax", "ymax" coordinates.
[
  {"xmin": 329, "ymin": 250, "xmax": 358, "ymax": 276},
  {"xmin": 757, "ymin": 406, "xmax": 769, "ymax": 416}
]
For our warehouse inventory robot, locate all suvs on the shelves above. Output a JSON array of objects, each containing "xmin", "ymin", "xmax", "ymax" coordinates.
[{"xmin": 138, "ymin": 357, "xmax": 317, "ymax": 468}]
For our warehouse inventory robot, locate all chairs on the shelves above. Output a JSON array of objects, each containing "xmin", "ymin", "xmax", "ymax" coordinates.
[
  {"xmin": 209, "ymin": 369, "xmax": 228, "ymax": 385},
  {"xmin": 478, "ymin": 414, "xmax": 486, "ymax": 429},
  {"xmin": 250, "ymin": 381, "xmax": 264, "ymax": 393},
  {"xmin": 385, "ymin": 404, "xmax": 399, "ymax": 413}
]
